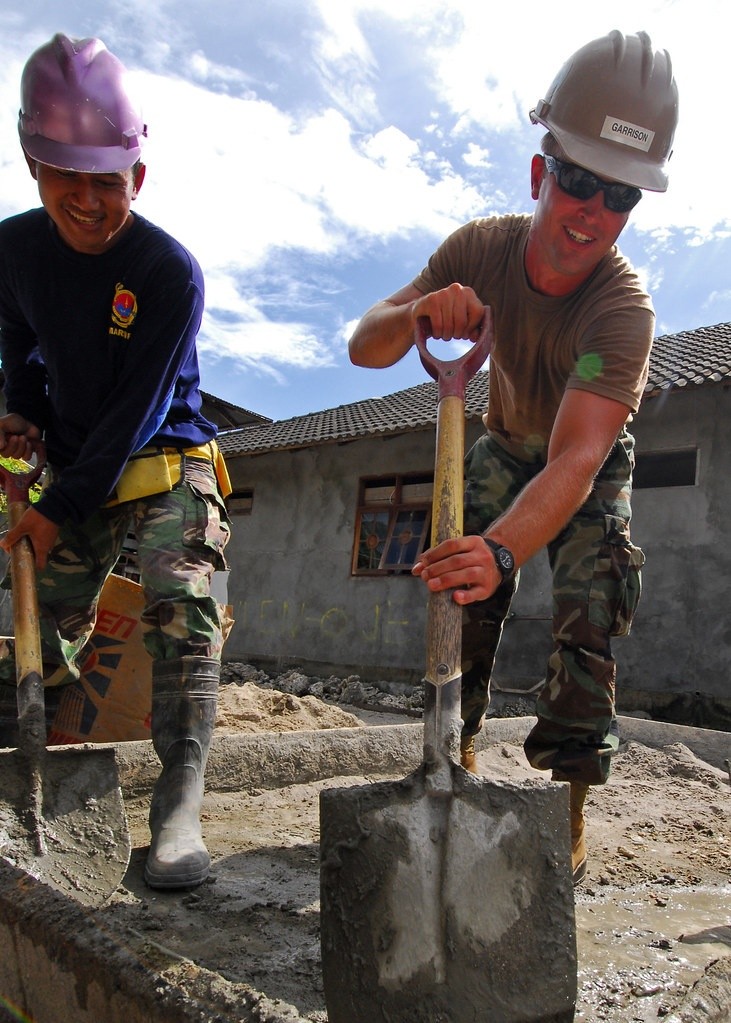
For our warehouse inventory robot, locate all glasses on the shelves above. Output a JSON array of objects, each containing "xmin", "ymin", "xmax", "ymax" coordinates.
[{"xmin": 542, "ymin": 153, "xmax": 642, "ymax": 212}]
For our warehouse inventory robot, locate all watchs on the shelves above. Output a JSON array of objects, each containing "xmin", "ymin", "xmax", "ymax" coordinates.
[{"xmin": 483, "ymin": 534, "xmax": 514, "ymax": 585}]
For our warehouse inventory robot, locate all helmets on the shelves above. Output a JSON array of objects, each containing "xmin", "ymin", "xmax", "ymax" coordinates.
[
  {"xmin": 529, "ymin": 29, "xmax": 679, "ymax": 193},
  {"xmin": 16, "ymin": 31, "xmax": 145, "ymax": 174}
]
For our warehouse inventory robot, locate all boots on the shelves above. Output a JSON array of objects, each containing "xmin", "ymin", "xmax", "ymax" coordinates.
[
  {"xmin": 549, "ymin": 774, "xmax": 588, "ymax": 885},
  {"xmin": 145, "ymin": 655, "xmax": 221, "ymax": 887},
  {"xmin": 460, "ymin": 733, "xmax": 477, "ymax": 775},
  {"xmin": 0, "ymin": 680, "xmax": 61, "ymax": 748}
]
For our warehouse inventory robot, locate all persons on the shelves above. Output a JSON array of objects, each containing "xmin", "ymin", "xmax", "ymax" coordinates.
[
  {"xmin": 0, "ymin": 32, "xmax": 232, "ymax": 888},
  {"xmin": 348, "ymin": 28, "xmax": 682, "ymax": 885}
]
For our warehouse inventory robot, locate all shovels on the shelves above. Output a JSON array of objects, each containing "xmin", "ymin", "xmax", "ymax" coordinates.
[
  {"xmin": 315, "ymin": 293, "xmax": 581, "ymax": 1023},
  {"xmin": 0, "ymin": 405, "xmax": 134, "ymax": 911}
]
